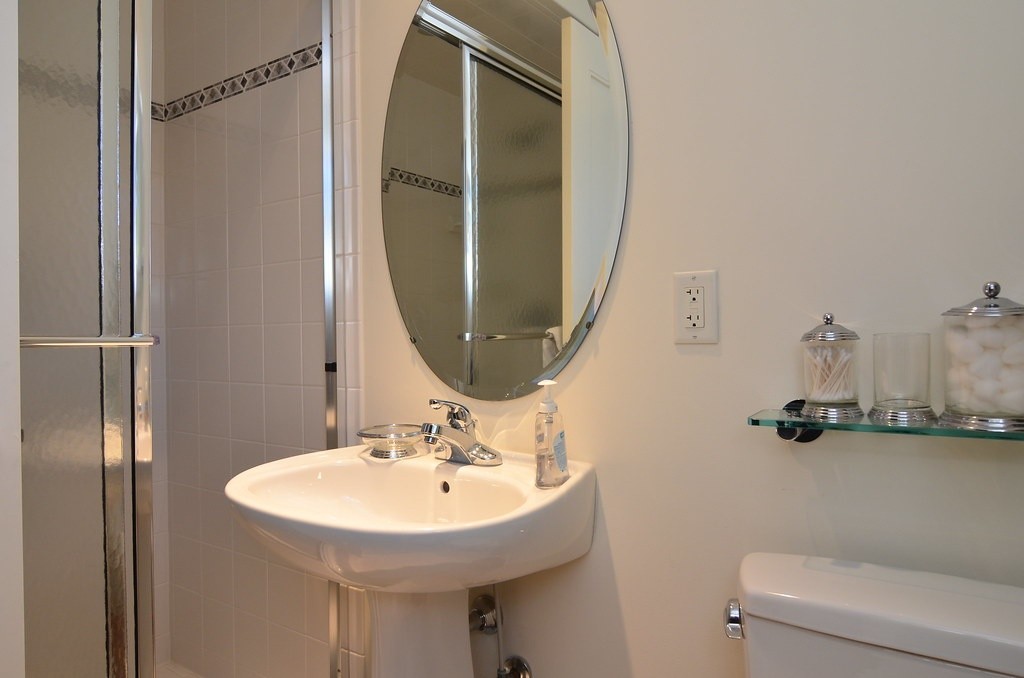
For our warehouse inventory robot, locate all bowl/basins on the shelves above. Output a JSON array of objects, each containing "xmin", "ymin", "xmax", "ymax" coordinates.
[{"xmin": 355, "ymin": 424, "xmax": 424, "ymax": 460}]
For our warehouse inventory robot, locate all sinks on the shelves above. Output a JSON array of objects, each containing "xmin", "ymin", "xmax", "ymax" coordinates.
[{"xmin": 224, "ymin": 442, "xmax": 596, "ymax": 595}]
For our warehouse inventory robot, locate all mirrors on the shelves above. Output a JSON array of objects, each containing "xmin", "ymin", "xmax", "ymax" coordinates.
[{"xmin": 380, "ymin": 0, "xmax": 629, "ymax": 401}]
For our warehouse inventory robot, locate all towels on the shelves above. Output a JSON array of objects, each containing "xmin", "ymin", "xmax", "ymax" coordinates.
[{"xmin": 541, "ymin": 323, "xmax": 563, "ymax": 369}]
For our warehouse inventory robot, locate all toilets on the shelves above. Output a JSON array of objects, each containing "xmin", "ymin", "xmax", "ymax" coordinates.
[{"xmin": 724, "ymin": 549, "xmax": 1023, "ymax": 678}]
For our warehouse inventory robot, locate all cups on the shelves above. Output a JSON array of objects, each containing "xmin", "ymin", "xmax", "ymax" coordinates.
[{"xmin": 870, "ymin": 332, "xmax": 937, "ymax": 425}]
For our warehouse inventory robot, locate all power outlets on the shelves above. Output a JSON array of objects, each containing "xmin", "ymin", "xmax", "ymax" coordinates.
[{"xmin": 675, "ymin": 270, "xmax": 720, "ymax": 343}]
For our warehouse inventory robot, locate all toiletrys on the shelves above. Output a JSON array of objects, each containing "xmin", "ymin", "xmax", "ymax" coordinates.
[{"xmin": 533, "ymin": 379, "xmax": 570, "ymax": 491}]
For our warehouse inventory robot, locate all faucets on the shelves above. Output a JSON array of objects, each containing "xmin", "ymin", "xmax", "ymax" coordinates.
[{"xmin": 421, "ymin": 397, "xmax": 503, "ymax": 466}]
